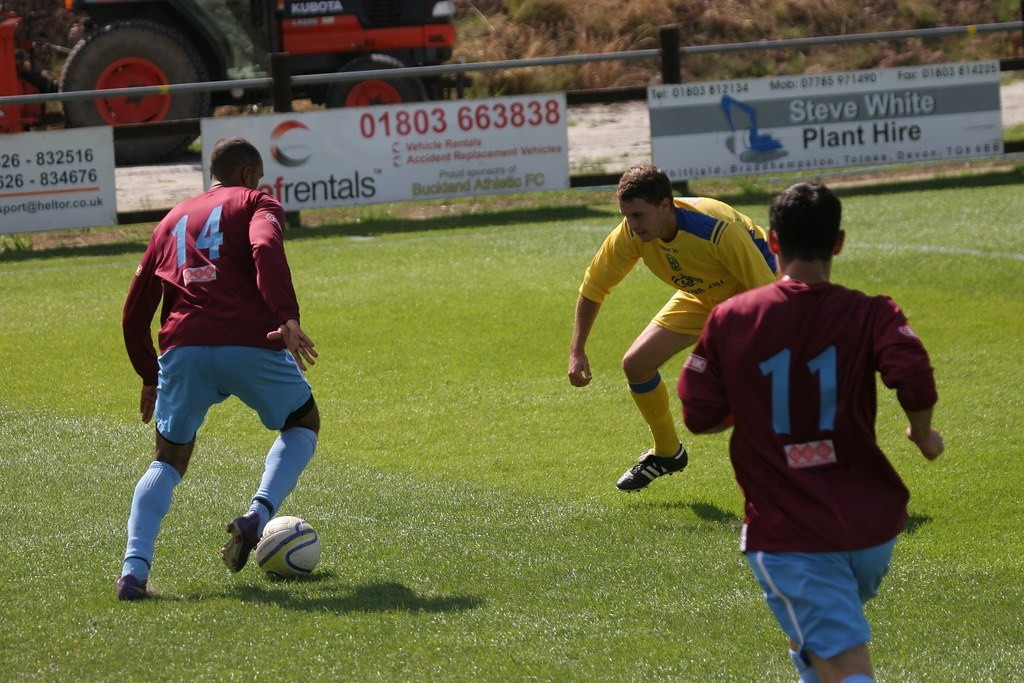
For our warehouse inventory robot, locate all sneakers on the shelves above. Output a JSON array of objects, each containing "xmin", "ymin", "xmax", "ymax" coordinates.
[
  {"xmin": 116, "ymin": 574, "xmax": 148, "ymax": 599},
  {"xmin": 221, "ymin": 512, "xmax": 259, "ymax": 572},
  {"xmin": 616, "ymin": 442, "xmax": 688, "ymax": 493}
]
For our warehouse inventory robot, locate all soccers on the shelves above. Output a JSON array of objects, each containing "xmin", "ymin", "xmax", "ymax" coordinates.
[{"xmin": 253, "ymin": 515, "xmax": 323, "ymax": 581}]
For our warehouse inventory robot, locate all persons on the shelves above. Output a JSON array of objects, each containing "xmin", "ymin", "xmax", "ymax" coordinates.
[
  {"xmin": 567, "ymin": 164, "xmax": 778, "ymax": 493},
  {"xmin": 676, "ymin": 183, "xmax": 944, "ymax": 683},
  {"xmin": 117, "ymin": 136, "xmax": 321, "ymax": 598}
]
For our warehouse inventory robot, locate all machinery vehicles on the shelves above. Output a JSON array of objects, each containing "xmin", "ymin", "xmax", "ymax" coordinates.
[{"xmin": 0, "ymin": 0, "xmax": 458, "ymax": 166}]
